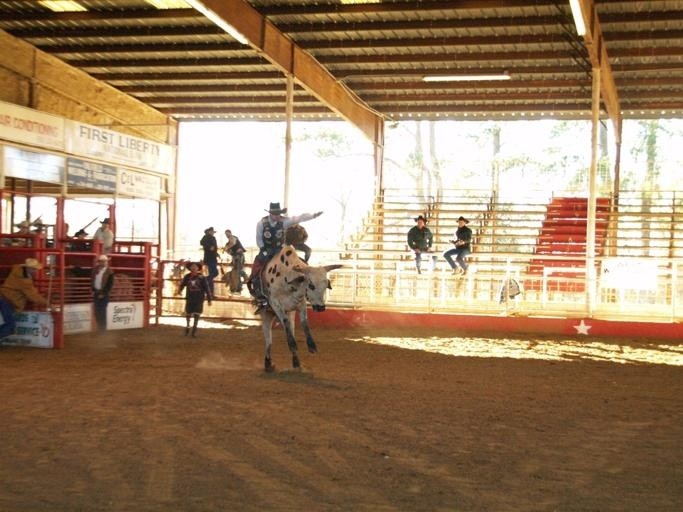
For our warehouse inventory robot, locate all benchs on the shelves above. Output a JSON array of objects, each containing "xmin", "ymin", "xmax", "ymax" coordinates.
[{"xmin": 332, "ymin": 182, "xmax": 683, "ymax": 312}]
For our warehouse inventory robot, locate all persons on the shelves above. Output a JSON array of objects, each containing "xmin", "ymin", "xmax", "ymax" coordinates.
[
  {"xmin": 442, "ymin": 215, "xmax": 472, "ymax": 275},
  {"xmin": 1, "ymin": 217, "xmax": 116, "ymax": 339},
  {"xmin": 407, "ymin": 215, "xmax": 442, "ymax": 274},
  {"xmin": 176, "ymin": 262, "xmax": 213, "ymax": 338},
  {"xmin": 285, "ymin": 222, "xmax": 312, "ymax": 264},
  {"xmin": 252, "ymin": 202, "xmax": 323, "ymax": 304},
  {"xmin": 224, "ymin": 230, "xmax": 246, "ymax": 296},
  {"xmin": 199, "ymin": 227, "xmax": 221, "ymax": 299}
]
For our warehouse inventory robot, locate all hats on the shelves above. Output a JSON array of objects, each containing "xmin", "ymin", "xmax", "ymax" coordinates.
[
  {"xmin": 99, "ymin": 218, "xmax": 112, "ymax": 225},
  {"xmin": 263, "ymin": 202, "xmax": 287, "ymax": 214},
  {"xmin": 74, "ymin": 229, "xmax": 88, "ymax": 237},
  {"xmin": 203, "ymin": 226, "xmax": 216, "ymax": 234},
  {"xmin": 185, "ymin": 261, "xmax": 202, "ymax": 271},
  {"xmin": 413, "ymin": 215, "xmax": 428, "ymax": 223},
  {"xmin": 455, "ymin": 216, "xmax": 470, "ymax": 224},
  {"xmin": 97, "ymin": 254, "xmax": 112, "ymax": 263},
  {"xmin": 23, "ymin": 258, "xmax": 43, "ymax": 271}
]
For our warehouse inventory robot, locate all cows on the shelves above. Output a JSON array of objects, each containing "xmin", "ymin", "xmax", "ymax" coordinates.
[{"xmin": 253, "ymin": 245, "xmax": 343, "ymax": 372}]
